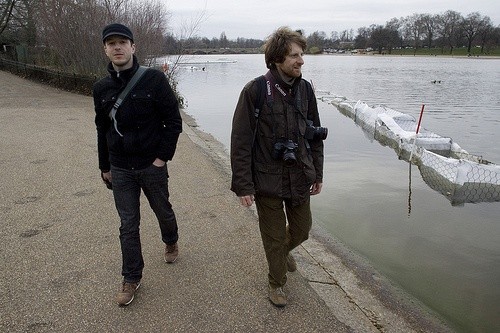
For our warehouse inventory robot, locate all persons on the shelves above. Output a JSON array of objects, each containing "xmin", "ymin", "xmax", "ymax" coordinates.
[
  {"xmin": 229, "ymin": 28, "xmax": 324, "ymax": 307},
  {"xmin": 92, "ymin": 23, "xmax": 183, "ymax": 306}
]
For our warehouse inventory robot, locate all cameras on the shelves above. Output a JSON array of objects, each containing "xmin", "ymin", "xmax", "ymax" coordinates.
[
  {"xmin": 272, "ymin": 140, "xmax": 299, "ymax": 167},
  {"xmin": 304, "ymin": 120, "xmax": 328, "ymax": 141}
]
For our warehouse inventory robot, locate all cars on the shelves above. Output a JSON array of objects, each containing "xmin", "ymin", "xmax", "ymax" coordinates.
[
  {"xmin": 324, "ymin": 49, "xmax": 358, "ymax": 54},
  {"xmin": 366, "ymin": 47, "xmax": 402, "ymax": 51},
  {"xmin": 476, "ymin": 45, "xmax": 481, "ymax": 48},
  {"xmin": 406, "ymin": 45, "xmax": 413, "ymax": 49}
]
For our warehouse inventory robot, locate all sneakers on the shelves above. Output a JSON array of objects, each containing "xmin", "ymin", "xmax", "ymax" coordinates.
[
  {"xmin": 116, "ymin": 277, "xmax": 142, "ymax": 305},
  {"xmin": 164, "ymin": 241, "xmax": 179, "ymax": 263},
  {"xmin": 286, "ymin": 251, "xmax": 296, "ymax": 272},
  {"xmin": 269, "ymin": 284, "xmax": 287, "ymax": 307}
]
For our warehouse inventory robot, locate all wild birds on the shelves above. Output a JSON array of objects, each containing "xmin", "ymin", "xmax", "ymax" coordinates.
[
  {"xmin": 431, "ymin": 79, "xmax": 442, "ymax": 84},
  {"xmin": 202, "ymin": 67, "xmax": 205, "ymax": 71}
]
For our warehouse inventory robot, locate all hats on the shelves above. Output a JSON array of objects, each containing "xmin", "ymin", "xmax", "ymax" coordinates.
[{"xmin": 102, "ymin": 23, "xmax": 134, "ymax": 43}]
